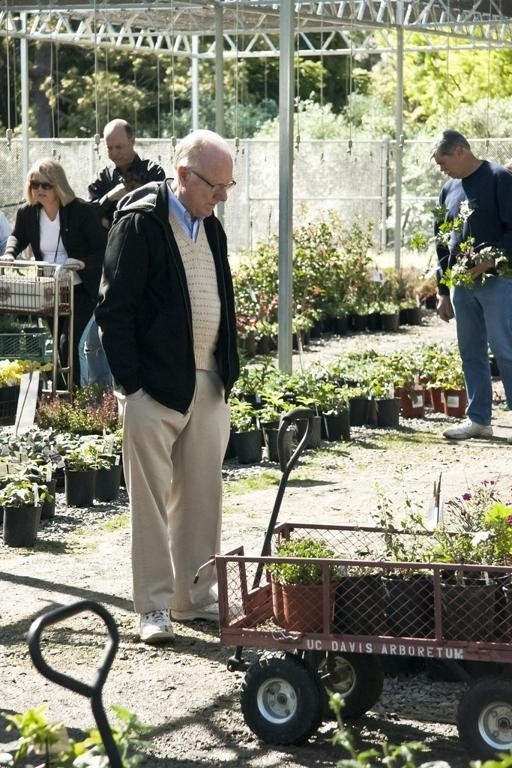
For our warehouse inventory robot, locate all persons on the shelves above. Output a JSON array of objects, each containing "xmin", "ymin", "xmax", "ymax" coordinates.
[
  {"xmin": 1, "ymin": 155, "xmax": 107, "ymax": 396},
  {"xmin": 91, "ymin": 127, "xmax": 243, "ymax": 645},
  {"xmin": 79, "ymin": 117, "xmax": 166, "ymax": 388},
  {"xmin": 427, "ymin": 128, "xmax": 512, "ymax": 445}
]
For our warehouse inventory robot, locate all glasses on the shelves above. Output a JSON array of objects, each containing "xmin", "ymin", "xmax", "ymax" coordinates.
[
  {"xmin": 191, "ymin": 170, "xmax": 236, "ymax": 192},
  {"xmin": 31, "ymin": 183, "xmax": 52, "ymax": 189}
]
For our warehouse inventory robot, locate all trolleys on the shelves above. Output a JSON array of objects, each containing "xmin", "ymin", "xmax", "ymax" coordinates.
[
  {"xmin": 1, "ymin": 257, "xmax": 89, "ymax": 405},
  {"xmin": 211, "ymin": 523, "xmax": 512, "ymax": 756}
]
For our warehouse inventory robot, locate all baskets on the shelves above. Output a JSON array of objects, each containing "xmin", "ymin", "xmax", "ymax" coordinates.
[{"xmin": 0, "ymin": 328, "xmax": 46, "ymax": 361}]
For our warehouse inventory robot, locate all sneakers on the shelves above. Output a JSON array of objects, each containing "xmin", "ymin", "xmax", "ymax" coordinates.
[
  {"xmin": 138, "ymin": 610, "xmax": 175, "ymax": 641},
  {"xmin": 443, "ymin": 420, "xmax": 492, "ymax": 439},
  {"xmin": 171, "ymin": 600, "xmax": 219, "ymax": 620}
]
